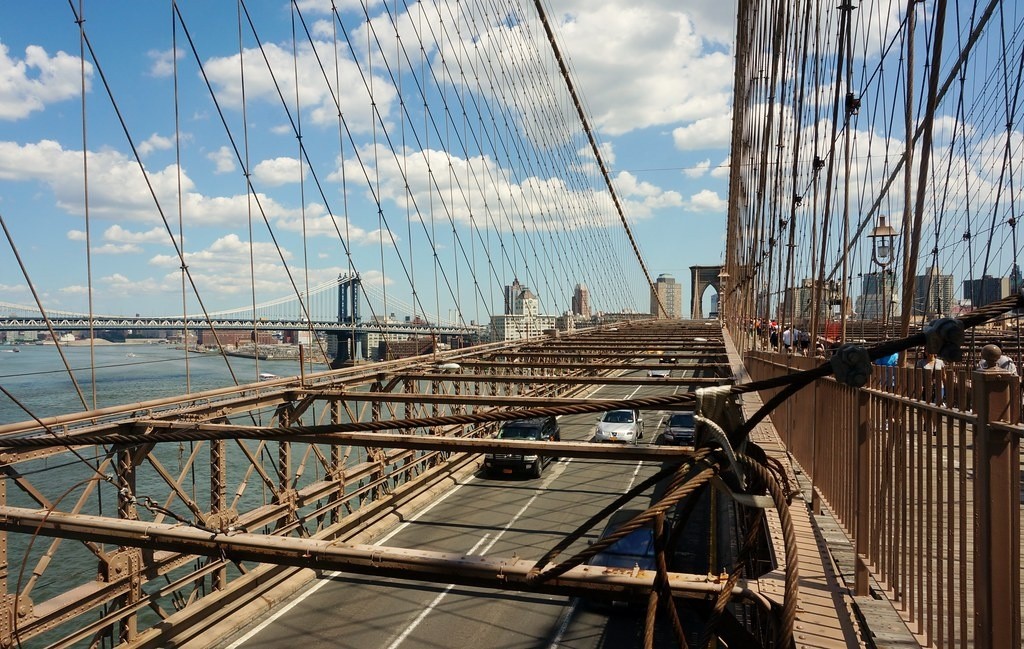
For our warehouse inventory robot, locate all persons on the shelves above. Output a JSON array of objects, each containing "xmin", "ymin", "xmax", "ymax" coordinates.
[
  {"xmin": 783, "ymin": 326, "xmax": 791, "ymax": 353},
  {"xmin": 769, "ymin": 319, "xmax": 778, "ymax": 351},
  {"xmin": 980, "ymin": 340, "xmax": 1016, "ymax": 376},
  {"xmin": 831, "ymin": 336, "xmax": 841, "ymax": 355},
  {"xmin": 918, "ymin": 347, "xmax": 945, "ymax": 435},
  {"xmin": 816, "ymin": 336, "xmax": 824, "ymax": 356},
  {"xmin": 750, "ymin": 318, "xmax": 765, "ymax": 340},
  {"xmin": 876, "ymin": 352, "xmax": 899, "ymax": 431},
  {"xmin": 793, "ymin": 325, "xmax": 809, "ymax": 356}
]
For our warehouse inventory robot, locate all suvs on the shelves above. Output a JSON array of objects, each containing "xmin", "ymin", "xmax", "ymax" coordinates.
[{"xmin": 483, "ymin": 416, "xmax": 562, "ymax": 479}]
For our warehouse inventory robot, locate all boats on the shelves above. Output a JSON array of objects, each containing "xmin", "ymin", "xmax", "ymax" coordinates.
[{"xmin": 259, "ymin": 373, "xmax": 278, "ymax": 382}]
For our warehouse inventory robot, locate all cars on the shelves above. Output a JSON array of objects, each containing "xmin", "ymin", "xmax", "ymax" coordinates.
[
  {"xmin": 581, "ymin": 508, "xmax": 667, "ymax": 606},
  {"xmin": 662, "ymin": 412, "xmax": 695, "ymax": 447},
  {"xmin": 646, "ymin": 368, "xmax": 672, "ymax": 378},
  {"xmin": 658, "ymin": 352, "xmax": 679, "ymax": 365},
  {"xmin": 596, "ymin": 407, "xmax": 645, "ymax": 446}
]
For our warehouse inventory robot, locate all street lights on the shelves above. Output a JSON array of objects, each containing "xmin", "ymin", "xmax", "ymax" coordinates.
[{"xmin": 867, "ymin": 214, "xmax": 901, "ymax": 342}]
